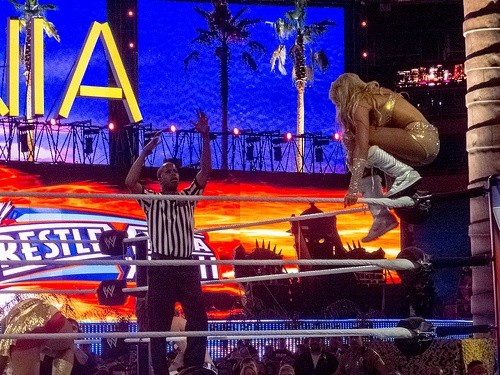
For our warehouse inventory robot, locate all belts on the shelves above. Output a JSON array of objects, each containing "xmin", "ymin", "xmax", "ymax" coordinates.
[{"xmin": 151, "ymin": 253, "xmax": 194, "ymax": 260}]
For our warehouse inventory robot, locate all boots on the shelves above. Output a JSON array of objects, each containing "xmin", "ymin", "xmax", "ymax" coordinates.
[
  {"xmin": 363, "ymin": 145, "xmax": 422, "ymax": 199},
  {"xmin": 358, "ymin": 176, "xmax": 398, "ymax": 242}
]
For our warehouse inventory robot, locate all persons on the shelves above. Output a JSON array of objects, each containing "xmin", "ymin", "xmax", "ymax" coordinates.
[
  {"xmin": 98, "ymin": 309, "xmax": 500, "ymax": 375},
  {"xmin": 79, "ymin": 342, "xmax": 113, "ymax": 375},
  {"xmin": 329, "ymin": 72, "xmax": 442, "ymax": 243},
  {"xmin": 39, "ymin": 316, "xmax": 94, "ymax": 375},
  {"xmin": 125, "ymin": 108, "xmax": 214, "ymax": 375},
  {"xmin": 0, "ymin": 298, "xmax": 76, "ymax": 375}
]
